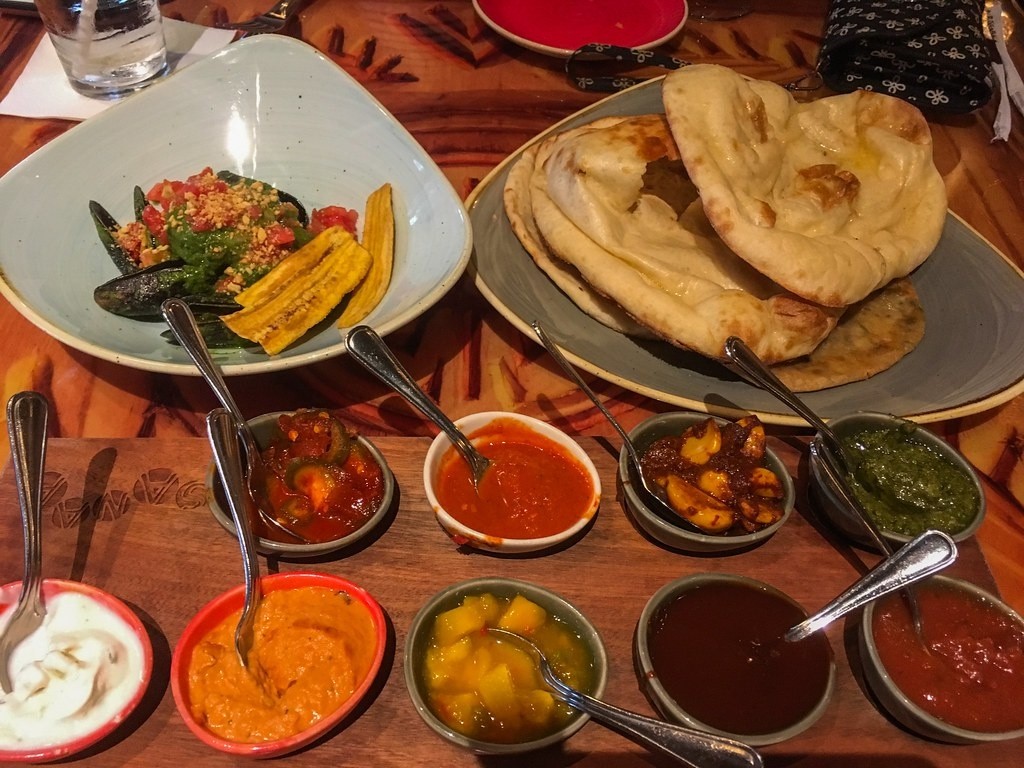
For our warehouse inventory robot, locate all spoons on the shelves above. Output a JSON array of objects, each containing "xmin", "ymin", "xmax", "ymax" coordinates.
[
  {"xmin": 809, "ymin": 436, "xmax": 937, "ymax": 655},
  {"xmin": 344, "ymin": 324, "xmax": 500, "ymax": 498},
  {"xmin": 204, "ymin": 408, "xmax": 274, "ymax": 703},
  {"xmin": 161, "ymin": 299, "xmax": 299, "ymax": 537},
  {"xmin": 487, "ymin": 629, "xmax": 760, "ymax": 767},
  {"xmin": 981, "ymin": 1, "xmax": 1013, "ymax": 40},
  {"xmin": 533, "ymin": 321, "xmax": 699, "ymax": 528},
  {"xmin": 0, "ymin": 390, "xmax": 51, "ymax": 695}
]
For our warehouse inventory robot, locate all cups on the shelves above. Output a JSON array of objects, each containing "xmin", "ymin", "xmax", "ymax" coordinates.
[{"xmin": 35, "ymin": 1, "xmax": 171, "ymax": 102}]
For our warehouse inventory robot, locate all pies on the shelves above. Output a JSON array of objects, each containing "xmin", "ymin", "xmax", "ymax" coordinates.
[{"xmin": 502, "ymin": 62, "xmax": 948, "ymax": 394}]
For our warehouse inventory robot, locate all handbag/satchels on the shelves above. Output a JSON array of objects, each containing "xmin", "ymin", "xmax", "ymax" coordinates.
[{"xmin": 783, "ymin": 0, "xmax": 994, "ymax": 113}]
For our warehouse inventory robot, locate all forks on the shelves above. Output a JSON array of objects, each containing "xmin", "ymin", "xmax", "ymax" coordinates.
[{"xmin": 214, "ymin": 1, "xmax": 313, "ymax": 33}]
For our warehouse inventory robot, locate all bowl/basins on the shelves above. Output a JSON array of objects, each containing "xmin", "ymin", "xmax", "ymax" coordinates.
[
  {"xmin": 1, "ymin": 33, "xmax": 474, "ymax": 376},
  {"xmin": 171, "ymin": 571, "xmax": 389, "ymax": 759},
  {"xmin": 858, "ymin": 574, "xmax": 1024, "ymax": 745},
  {"xmin": 404, "ymin": 578, "xmax": 607, "ymax": 755},
  {"xmin": 635, "ymin": 571, "xmax": 837, "ymax": 746},
  {"xmin": 422, "ymin": 411, "xmax": 601, "ymax": 552},
  {"xmin": 807, "ymin": 411, "xmax": 986, "ymax": 542},
  {"xmin": 619, "ymin": 411, "xmax": 795, "ymax": 553},
  {"xmin": 1, "ymin": 578, "xmax": 153, "ymax": 762}
]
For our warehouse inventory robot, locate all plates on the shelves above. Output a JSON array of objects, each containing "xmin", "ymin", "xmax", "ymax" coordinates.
[
  {"xmin": 463, "ymin": 68, "xmax": 1024, "ymax": 426},
  {"xmin": 204, "ymin": 412, "xmax": 395, "ymax": 558},
  {"xmin": 472, "ymin": 1, "xmax": 688, "ymax": 59}
]
